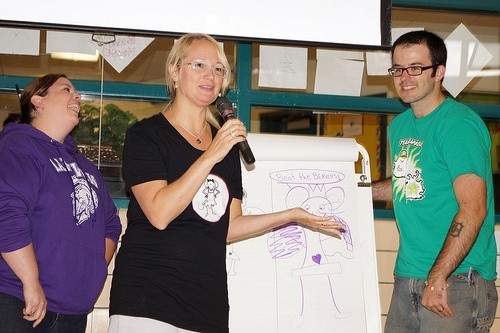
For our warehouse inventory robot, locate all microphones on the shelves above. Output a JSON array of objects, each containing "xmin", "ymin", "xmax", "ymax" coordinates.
[{"xmin": 215, "ymin": 98, "xmax": 255, "ymax": 165}]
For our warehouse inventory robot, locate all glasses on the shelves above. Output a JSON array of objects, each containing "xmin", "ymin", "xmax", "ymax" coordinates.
[
  {"xmin": 177, "ymin": 60, "xmax": 227, "ymax": 77},
  {"xmin": 388, "ymin": 63, "xmax": 439, "ymax": 77}
]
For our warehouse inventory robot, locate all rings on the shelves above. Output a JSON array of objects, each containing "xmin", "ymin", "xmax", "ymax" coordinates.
[
  {"xmin": 321, "ymin": 221, "xmax": 324, "ymax": 226},
  {"xmin": 29, "ymin": 311, "xmax": 34, "ymax": 316},
  {"xmin": 437, "ymin": 307, "xmax": 445, "ymax": 312}
]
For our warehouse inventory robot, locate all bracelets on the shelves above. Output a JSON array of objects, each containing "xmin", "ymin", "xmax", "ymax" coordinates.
[{"xmin": 425, "ymin": 280, "xmax": 449, "ymax": 292}]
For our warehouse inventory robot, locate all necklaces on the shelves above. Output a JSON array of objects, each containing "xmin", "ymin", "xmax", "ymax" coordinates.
[{"xmin": 168, "ymin": 105, "xmax": 208, "ymax": 144}]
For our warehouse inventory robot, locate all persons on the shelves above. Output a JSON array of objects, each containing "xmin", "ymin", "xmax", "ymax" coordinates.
[
  {"xmin": 0, "ymin": 73, "xmax": 123, "ymax": 333},
  {"xmin": 372, "ymin": 28, "xmax": 498, "ymax": 333},
  {"xmin": 107, "ymin": 31, "xmax": 343, "ymax": 333}
]
